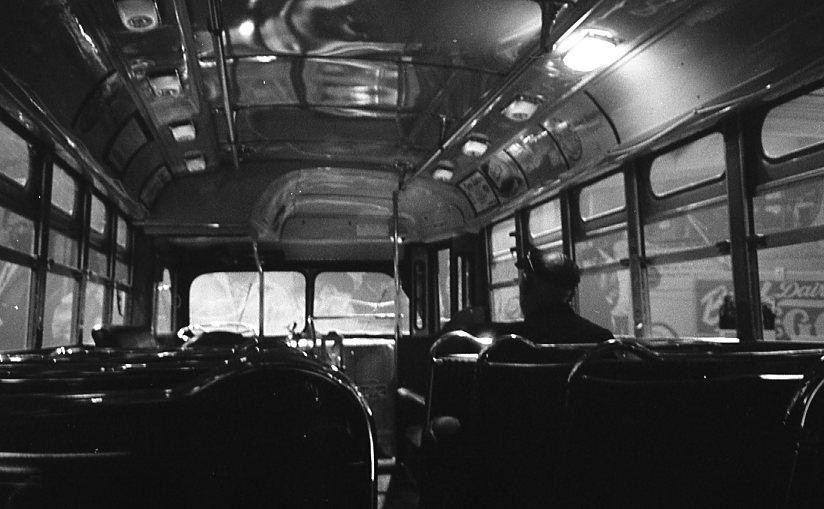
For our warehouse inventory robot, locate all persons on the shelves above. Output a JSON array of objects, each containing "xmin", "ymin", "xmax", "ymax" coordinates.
[{"xmin": 513, "ymin": 250, "xmax": 616, "ymax": 344}]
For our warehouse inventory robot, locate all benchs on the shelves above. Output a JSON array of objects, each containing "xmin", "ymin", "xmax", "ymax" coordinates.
[{"xmin": 3, "ymin": 329, "xmax": 824, "ymax": 509}]
